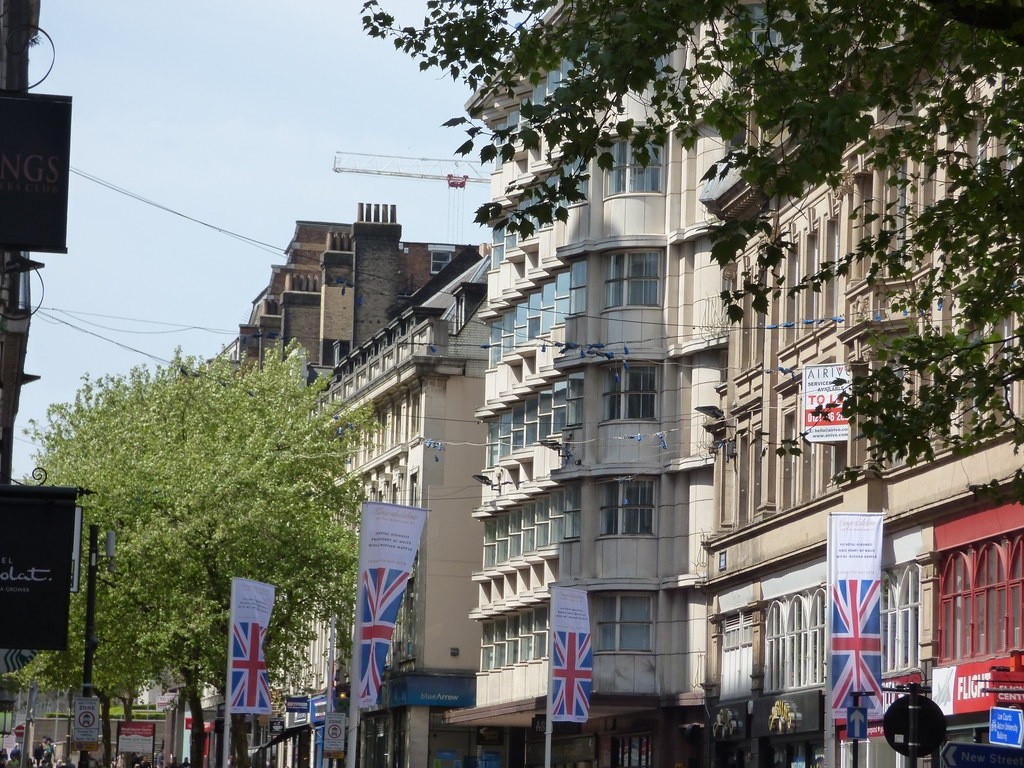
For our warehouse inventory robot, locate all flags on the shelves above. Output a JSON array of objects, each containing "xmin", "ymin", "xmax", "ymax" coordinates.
[
  {"xmin": 361, "ymin": 503, "xmax": 427, "ymax": 706},
  {"xmin": 832, "ymin": 516, "xmax": 883, "ymax": 739},
  {"xmin": 552, "ymin": 588, "xmax": 592, "ymax": 723},
  {"xmin": 231, "ymin": 579, "xmax": 275, "ymax": 713}
]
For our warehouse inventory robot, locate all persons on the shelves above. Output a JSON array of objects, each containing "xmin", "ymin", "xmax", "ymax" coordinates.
[{"xmin": 0, "ymin": 736, "xmax": 237, "ymax": 768}]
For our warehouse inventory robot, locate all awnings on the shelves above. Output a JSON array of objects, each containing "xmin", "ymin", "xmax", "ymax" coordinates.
[{"xmin": 264, "ymin": 724, "xmax": 310, "ymax": 748}]
